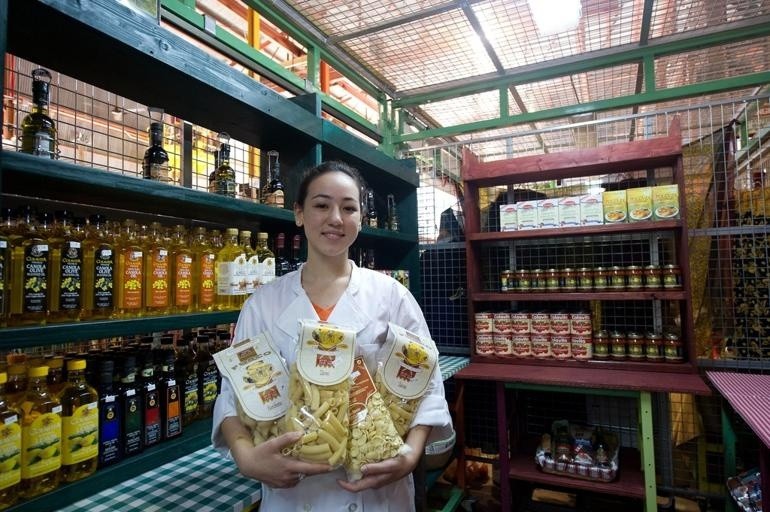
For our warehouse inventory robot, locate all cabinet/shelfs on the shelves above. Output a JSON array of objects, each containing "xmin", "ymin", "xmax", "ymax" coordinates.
[
  {"xmin": 461, "ymin": 116, "xmax": 698, "ymax": 372},
  {"xmin": 0, "ymin": 0, "xmax": 419, "ymax": 510}
]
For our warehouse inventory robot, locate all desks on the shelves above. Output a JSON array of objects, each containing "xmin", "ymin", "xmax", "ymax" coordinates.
[
  {"xmin": 55, "ymin": 356, "xmax": 471, "ymax": 512},
  {"xmin": 449, "ymin": 363, "xmax": 717, "ymax": 512},
  {"xmin": 701, "ymin": 363, "xmax": 769, "ymax": 512}
]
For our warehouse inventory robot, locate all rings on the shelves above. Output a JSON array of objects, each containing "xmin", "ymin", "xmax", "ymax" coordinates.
[{"xmin": 298, "ymin": 473, "xmax": 307, "ymax": 481}]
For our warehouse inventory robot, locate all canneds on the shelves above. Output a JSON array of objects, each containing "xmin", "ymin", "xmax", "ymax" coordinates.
[
  {"xmin": 592, "ymin": 329, "xmax": 684, "ymax": 364},
  {"xmin": 474, "ymin": 311, "xmax": 592, "ymax": 361},
  {"xmin": 501, "ymin": 264, "xmax": 681, "ymax": 294}
]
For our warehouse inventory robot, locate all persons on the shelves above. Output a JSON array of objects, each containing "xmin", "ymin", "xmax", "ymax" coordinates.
[{"xmin": 209, "ymin": 159, "xmax": 451, "ymax": 512}]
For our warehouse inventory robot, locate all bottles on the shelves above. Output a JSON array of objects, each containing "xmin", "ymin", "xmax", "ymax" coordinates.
[
  {"xmin": 143, "ymin": 123, "xmax": 169, "ymax": 184},
  {"xmin": 266, "ymin": 163, "xmax": 285, "ymax": 209},
  {"xmin": 215, "ymin": 143, "xmax": 235, "ymax": 198},
  {"xmin": 389, "ymin": 204, "xmax": 400, "ymax": 232},
  {"xmin": 0, "ymin": 322, "xmax": 236, "ymax": 512},
  {"xmin": 21, "ymin": 68, "xmax": 55, "ymax": 159},
  {"xmin": 209, "ymin": 151, "xmax": 227, "ymax": 196},
  {"xmin": 0, "ymin": 203, "xmax": 306, "ymax": 330}
]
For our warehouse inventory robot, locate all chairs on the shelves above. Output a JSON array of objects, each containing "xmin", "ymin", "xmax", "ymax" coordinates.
[{"xmin": 424, "ymin": 436, "xmax": 469, "ymax": 512}]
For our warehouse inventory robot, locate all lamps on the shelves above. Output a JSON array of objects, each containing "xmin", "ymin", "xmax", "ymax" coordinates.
[{"xmin": 527, "ymin": 0, "xmax": 582, "ymax": 37}]
